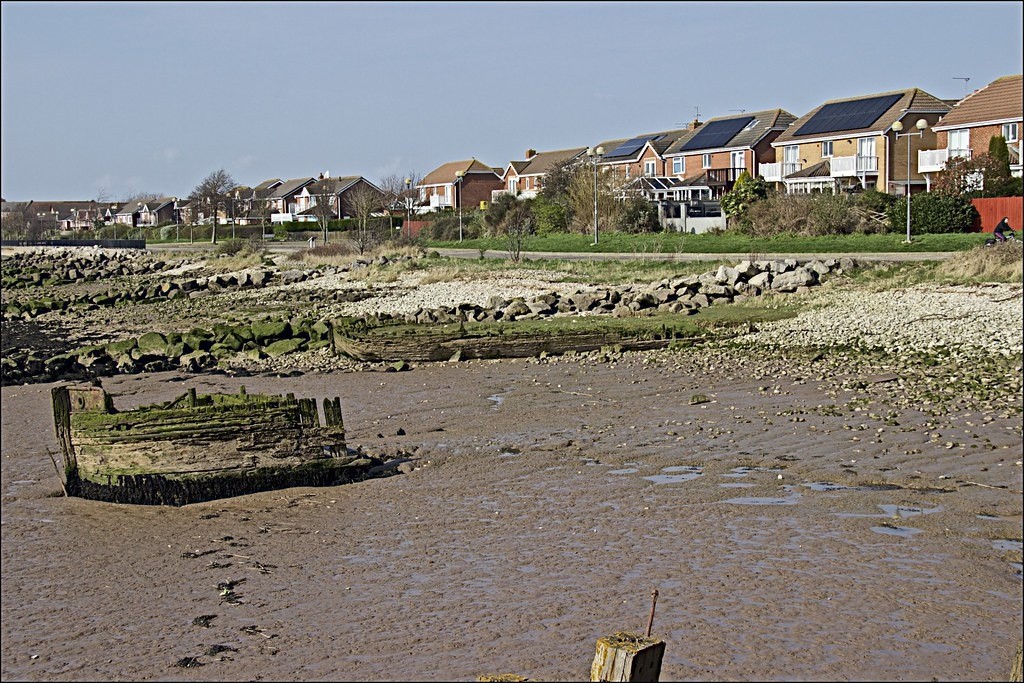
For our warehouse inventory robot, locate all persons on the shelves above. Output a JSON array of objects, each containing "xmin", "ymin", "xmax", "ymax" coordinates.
[{"xmin": 993, "ymin": 217, "xmax": 1016, "ymax": 244}]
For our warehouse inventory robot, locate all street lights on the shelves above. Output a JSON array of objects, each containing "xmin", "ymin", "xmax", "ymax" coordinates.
[
  {"xmin": 405, "ymin": 178, "xmax": 412, "ymax": 237},
  {"xmin": 226, "ymin": 191, "xmax": 240, "ymax": 238},
  {"xmin": 585, "ymin": 146, "xmax": 605, "ymax": 247},
  {"xmin": 891, "ymin": 118, "xmax": 928, "ymax": 245},
  {"xmin": 36, "ymin": 209, "xmax": 76, "ymax": 240},
  {"xmin": 322, "ymin": 186, "xmax": 331, "ymax": 244},
  {"xmin": 454, "ymin": 170, "xmax": 464, "ymax": 244},
  {"xmin": 172, "ymin": 196, "xmax": 182, "ymax": 243},
  {"xmin": 111, "ymin": 203, "xmax": 118, "ymax": 239}
]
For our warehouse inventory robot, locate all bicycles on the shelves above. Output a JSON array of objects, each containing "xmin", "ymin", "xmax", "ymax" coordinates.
[{"xmin": 984, "ymin": 233, "xmax": 1023, "ymax": 249}]
